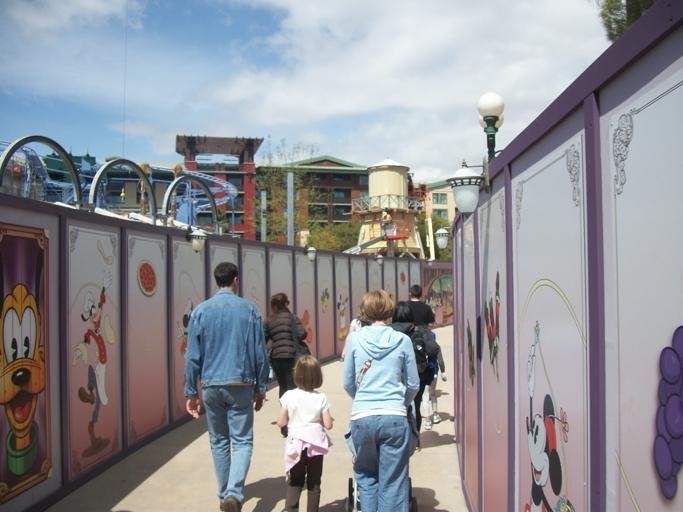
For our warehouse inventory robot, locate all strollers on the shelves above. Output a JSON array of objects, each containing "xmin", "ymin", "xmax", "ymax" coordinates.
[{"xmin": 345, "ymin": 405, "xmax": 418, "ymax": 512}]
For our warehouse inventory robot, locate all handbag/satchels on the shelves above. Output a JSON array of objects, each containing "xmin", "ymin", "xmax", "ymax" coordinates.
[{"xmin": 292, "ymin": 328, "xmax": 311, "ymax": 355}]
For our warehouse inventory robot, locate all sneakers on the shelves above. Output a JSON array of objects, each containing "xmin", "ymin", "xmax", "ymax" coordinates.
[
  {"xmin": 425, "ymin": 421, "xmax": 432, "ymax": 429},
  {"xmin": 432, "ymin": 415, "xmax": 442, "ymax": 423},
  {"xmin": 220, "ymin": 496, "xmax": 242, "ymax": 512}
]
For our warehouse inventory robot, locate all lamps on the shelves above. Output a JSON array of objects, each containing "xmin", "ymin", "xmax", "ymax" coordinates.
[
  {"xmin": 188, "ymin": 230, "xmax": 207, "ymax": 253},
  {"xmin": 434, "ymin": 226, "xmax": 454, "ymax": 250},
  {"xmin": 305, "ymin": 244, "xmax": 317, "ymax": 261},
  {"xmin": 446, "ymin": 157, "xmax": 489, "ymax": 215}
]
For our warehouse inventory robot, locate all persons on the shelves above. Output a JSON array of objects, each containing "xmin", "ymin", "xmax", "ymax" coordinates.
[
  {"xmin": 344, "ymin": 290, "xmax": 421, "ymax": 511},
  {"xmin": 341, "ymin": 308, "xmax": 373, "ymax": 361},
  {"xmin": 382, "ymin": 211, "xmax": 393, "ymax": 221},
  {"xmin": 388, "ymin": 284, "xmax": 446, "ymax": 448},
  {"xmin": 183, "ymin": 262, "xmax": 269, "ymax": 512},
  {"xmin": 277, "ymin": 356, "xmax": 333, "ymax": 512},
  {"xmin": 262, "ymin": 293, "xmax": 309, "ymax": 435}
]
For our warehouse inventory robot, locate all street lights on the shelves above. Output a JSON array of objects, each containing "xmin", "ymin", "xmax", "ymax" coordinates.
[{"xmin": 477, "ymin": 92, "xmax": 504, "ymax": 162}]
